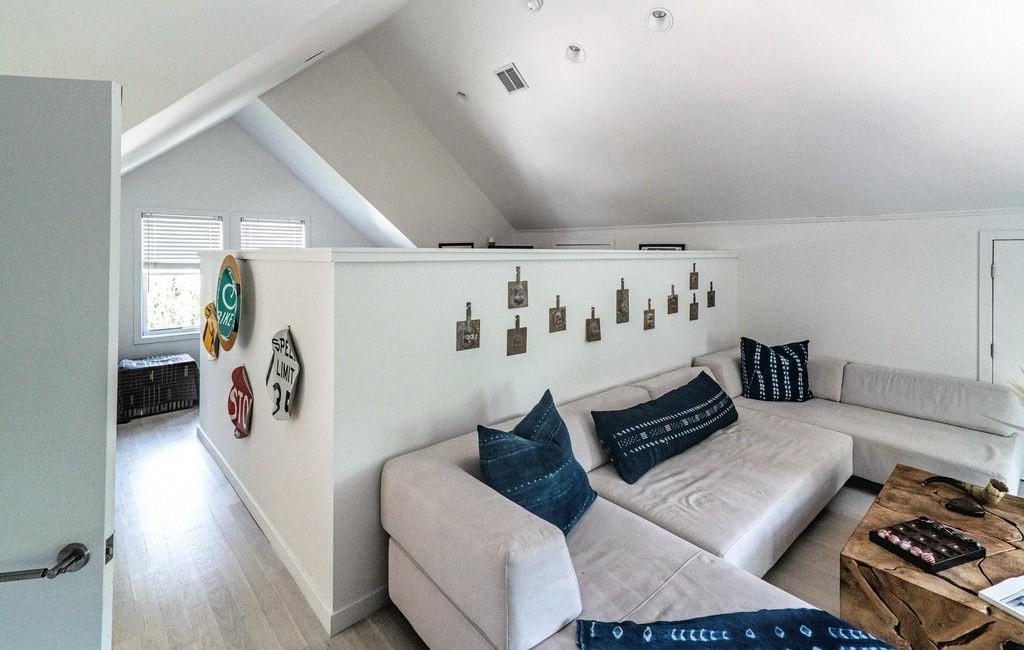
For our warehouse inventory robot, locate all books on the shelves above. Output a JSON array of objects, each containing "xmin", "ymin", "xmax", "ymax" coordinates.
[{"xmin": 978, "ymin": 575, "xmax": 1024, "ymax": 622}]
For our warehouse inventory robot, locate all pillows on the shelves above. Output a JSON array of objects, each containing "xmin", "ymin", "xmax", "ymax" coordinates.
[
  {"xmin": 739, "ymin": 336, "xmax": 814, "ymax": 403},
  {"xmin": 592, "ymin": 370, "xmax": 739, "ymax": 484},
  {"xmin": 477, "ymin": 389, "xmax": 598, "ymax": 537}
]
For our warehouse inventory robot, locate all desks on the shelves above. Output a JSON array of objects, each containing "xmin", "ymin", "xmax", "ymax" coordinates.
[{"xmin": 116, "ymin": 353, "xmax": 200, "ymax": 424}]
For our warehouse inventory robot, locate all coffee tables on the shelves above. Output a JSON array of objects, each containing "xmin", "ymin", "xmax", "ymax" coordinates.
[{"xmin": 839, "ymin": 463, "xmax": 1024, "ymax": 650}]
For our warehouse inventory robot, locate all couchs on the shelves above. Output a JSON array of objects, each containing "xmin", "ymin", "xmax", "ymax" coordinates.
[{"xmin": 378, "ymin": 347, "xmax": 1023, "ymax": 650}]
